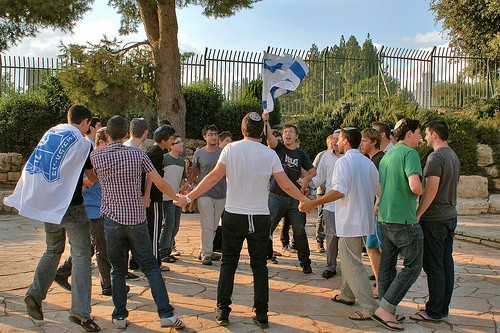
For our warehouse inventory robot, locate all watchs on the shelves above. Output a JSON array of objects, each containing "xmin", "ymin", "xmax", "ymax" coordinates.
[{"xmin": 186, "ymin": 195, "xmax": 192, "ymax": 203}]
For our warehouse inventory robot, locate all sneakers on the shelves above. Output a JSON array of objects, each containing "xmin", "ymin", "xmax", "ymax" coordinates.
[
  {"xmin": 113, "ymin": 318, "xmax": 126, "ymax": 329},
  {"xmin": 160, "ymin": 315, "xmax": 185, "ymax": 328}
]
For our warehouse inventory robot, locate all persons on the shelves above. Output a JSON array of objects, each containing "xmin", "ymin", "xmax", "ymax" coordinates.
[{"xmin": 25, "ymin": 106, "xmax": 460, "ymax": 332}]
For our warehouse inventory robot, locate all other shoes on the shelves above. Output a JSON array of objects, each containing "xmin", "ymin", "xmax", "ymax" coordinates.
[
  {"xmin": 368, "ymin": 274, "xmax": 375, "ymax": 280},
  {"xmin": 322, "ymin": 270, "xmax": 337, "ymax": 278},
  {"xmin": 102, "ymin": 286, "xmax": 131, "ymax": 296},
  {"xmin": 215, "ymin": 315, "xmax": 232, "ymax": 326},
  {"xmin": 282, "ymin": 245, "xmax": 298, "ymax": 253},
  {"xmin": 198, "ymin": 252, "xmax": 221, "ymax": 260},
  {"xmin": 267, "ymin": 258, "xmax": 278, "ymax": 264},
  {"xmin": 252, "ymin": 316, "xmax": 268, "ymax": 328},
  {"xmin": 202, "ymin": 256, "xmax": 212, "ymax": 265},
  {"xmin": 373, "ymin": 294, "xmax": 379, "ymax": 299},
  {"xmin": 24, "ymin": 294, "xmax": 44, "ymax": 320},
  {"xmin": 317, "ymin": 246, "xmax": 325, "ymax": 253},
  {"xmin": 125, "ymin": 272, "xmax": 140, "ymax": 281},
  {"xmin": 170, "ymin": 249, "xmax": 181, "ymax": 256},
  {"xmin": 54, "ymin": 274, "xmax": 71, "ymax": 291},
  {"xmin": 303, "ymin": 266, "xmax": 312, "ymax": 274},
  {"xmin": 160, "ymin": 256, "xmax": 179, "ymax": 263},
  {"xmin": 69, "ymin": 315, "xmax": 101, "ymax": 332}
]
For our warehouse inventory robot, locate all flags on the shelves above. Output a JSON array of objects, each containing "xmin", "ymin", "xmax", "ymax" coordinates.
[{"xmin": 261, "ymin": 52, "xmax": 309, "ymax": 117}]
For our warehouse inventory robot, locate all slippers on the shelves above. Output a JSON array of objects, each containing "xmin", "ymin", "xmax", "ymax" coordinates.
[
  {"xmin": 348, "ymin": 311, "xmax": 373, "ymax": 320},
  {"xmin": 331, "ymin": 294, "xmax": 355, "ymax": 306},
  {"xmin": 392, "ymin": 314, "xmax": 406, "ymax": 321},
  {"xmin": 409, "ymin": 309, "xmax": 442, "ymax": 324},
  {"xmin": 371, "ymin": 315, "xmax": 405, "ymax": 332}
]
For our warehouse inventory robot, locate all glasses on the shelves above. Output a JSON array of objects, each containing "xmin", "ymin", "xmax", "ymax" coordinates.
[{"xmin": 173, "ymin": 139, "xmax": 184, "ymax": 145}]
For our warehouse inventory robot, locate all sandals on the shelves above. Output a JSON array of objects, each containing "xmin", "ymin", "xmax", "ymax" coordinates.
[{"xmin": 159, "ymin": 265, "xmax": 170, "ymax": 271}]
